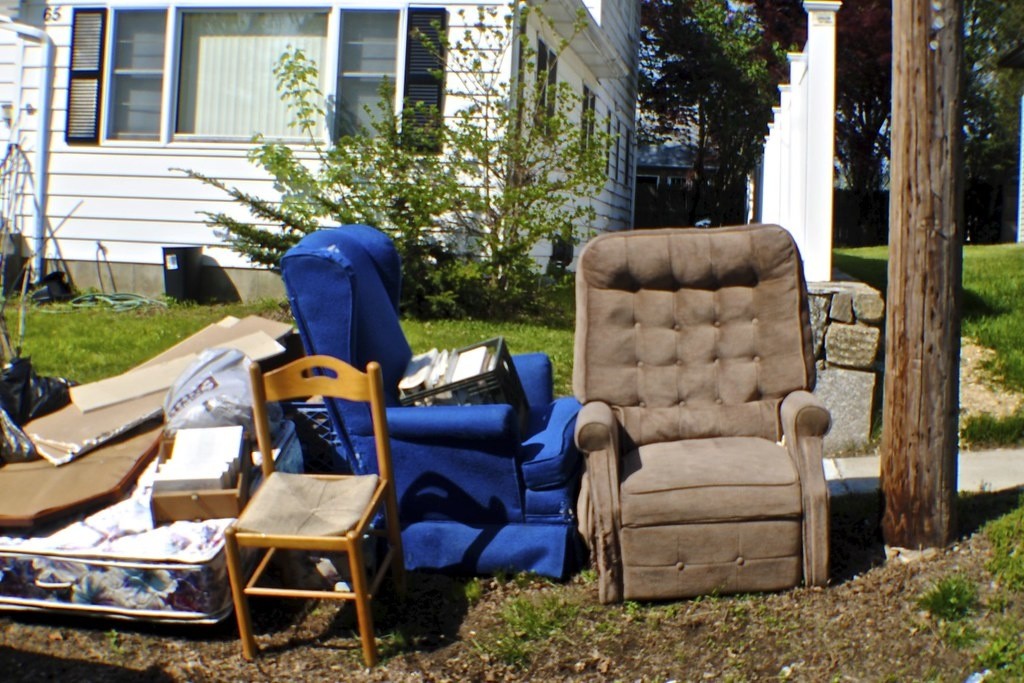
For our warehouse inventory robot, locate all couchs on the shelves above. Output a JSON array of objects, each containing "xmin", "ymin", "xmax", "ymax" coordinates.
[
  {"xmin": 570, "ymin": 223, "xmax": 834, "ymax": 604},
  {"xmin": 280, "ymin": 222, "xmax": 582, "ymax": 576}
]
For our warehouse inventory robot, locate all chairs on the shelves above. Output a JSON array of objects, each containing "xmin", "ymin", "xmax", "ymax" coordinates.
[{"xmin": 221, "ymin": 353, "xmax": 402, "ymax": 669}]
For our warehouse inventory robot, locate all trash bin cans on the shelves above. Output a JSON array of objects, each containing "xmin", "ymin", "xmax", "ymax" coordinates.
[{"xmin": 163, "ymin": 247, "xmax": 203, "ymax": 305}]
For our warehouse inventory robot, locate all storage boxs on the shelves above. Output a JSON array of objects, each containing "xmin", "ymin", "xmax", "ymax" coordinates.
[
  {"xmin": 149, "ymin": 440, "xmax": 243, "ymax": 521},
  {"xmin": 399, "ymin": 333, "xmax": 534, "ymax": 428}
]
